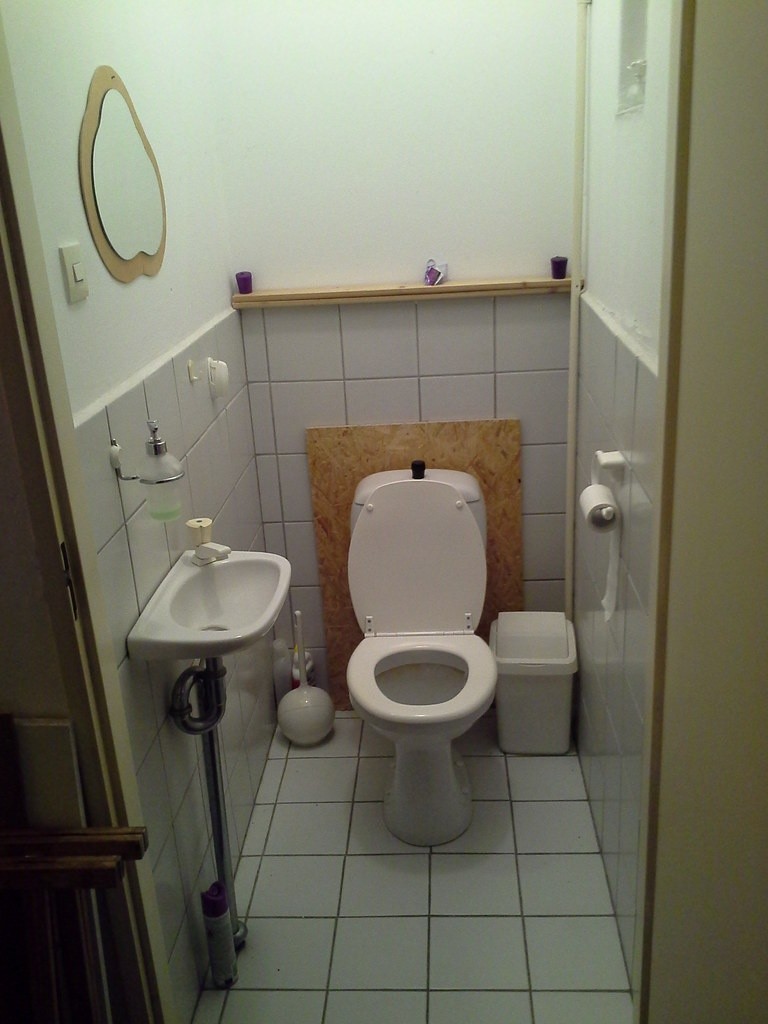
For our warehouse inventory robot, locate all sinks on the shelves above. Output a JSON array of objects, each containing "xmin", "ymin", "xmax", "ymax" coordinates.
[{"xmin": 127, "ymin": 550, "xmax": 291, "ymax": 663}]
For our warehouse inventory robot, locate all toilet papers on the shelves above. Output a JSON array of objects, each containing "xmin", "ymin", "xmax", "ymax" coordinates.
[{"xmin": 579, "ymin": 480, "xmax": 622, "ymax": 624}]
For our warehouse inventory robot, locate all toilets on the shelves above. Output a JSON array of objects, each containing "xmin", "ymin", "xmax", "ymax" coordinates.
[{"xmin": 343, "ymin": 469, "xmax": 490, "ymax": 848}]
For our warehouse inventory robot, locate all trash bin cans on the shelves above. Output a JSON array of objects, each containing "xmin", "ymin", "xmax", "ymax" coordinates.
[{"xmin": 489, "ymin": 611, "xmax": 579, "ymax": 755}]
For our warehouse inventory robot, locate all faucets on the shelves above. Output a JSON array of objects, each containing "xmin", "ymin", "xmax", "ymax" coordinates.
[{"xmin": 187, "ymin": 515, "xmax": 231, "ymax": 570}]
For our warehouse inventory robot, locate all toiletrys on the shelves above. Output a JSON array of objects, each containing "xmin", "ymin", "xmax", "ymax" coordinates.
[{"xmin": 138, "ymin": 416, "xmax": 185, "ymax": 522}]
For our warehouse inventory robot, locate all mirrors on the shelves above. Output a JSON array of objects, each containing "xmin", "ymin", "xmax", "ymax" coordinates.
[{"xmin": 92, "ymin": 89, "xmax": 163, "ymax": 261}]
